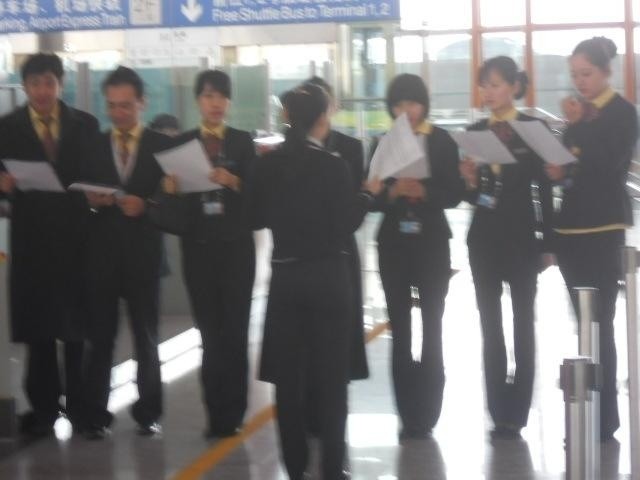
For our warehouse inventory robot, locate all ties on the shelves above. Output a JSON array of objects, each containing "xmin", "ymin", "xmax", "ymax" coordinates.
[
  {"xmin": 39, "ymin": 118, "xmax": 53, "ymax": 155},
  {"xmin": 492, "ymin": 122, "xmax": 511, "ymax": 145},
  {"xmin": 205, "ymin": 132, "xmax": 219, "ymax": 165},
  {"xmin": 118, "ymin": 133, "xmax": 131, "ymax": 168}
]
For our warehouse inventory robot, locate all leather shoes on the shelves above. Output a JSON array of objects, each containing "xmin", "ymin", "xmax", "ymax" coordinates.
[
  {"xmin": 24, "ymin": 422, "xmax": 109, "ymax": 440},
  {"xmin": 136, "ymin": 423, "xmax": 161, "ymax": 436}
]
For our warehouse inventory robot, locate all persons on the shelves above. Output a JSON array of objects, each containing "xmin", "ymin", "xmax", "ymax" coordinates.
[
  {"xmin": 458, "ymin": 54, "xmax": 556, "ymax": 435},
  {"xmin": 243, "ymin": 84, "xmax": 384, "ymax": 480},
  {"xmin": 71, "ymin": 65, "xmax": 185, "ymax": 443},
  {"xmin": 149, "ymin": 112, "xmax": 184, "ymax": 138},
  {"xmin": 1, "ymin": 50, "xmax": 108, "ymax": 441},
  {"xmin": 546, "ymin": 35, "xmax": 640, "ymax": 442},
  {"xmin": 287, "ymin": 75, "xmax": 366, "ymax": 439},
  {"xmin": 161, "ymin": 69, "xmax": 257, "ymax": 436},
  {"xmin": 368, "ymin": 71, "xmax": 463, "ymax": 436},
  {"xmin": 280, "ymin": 90, "xmax": 295, "ymax": 124}
]
